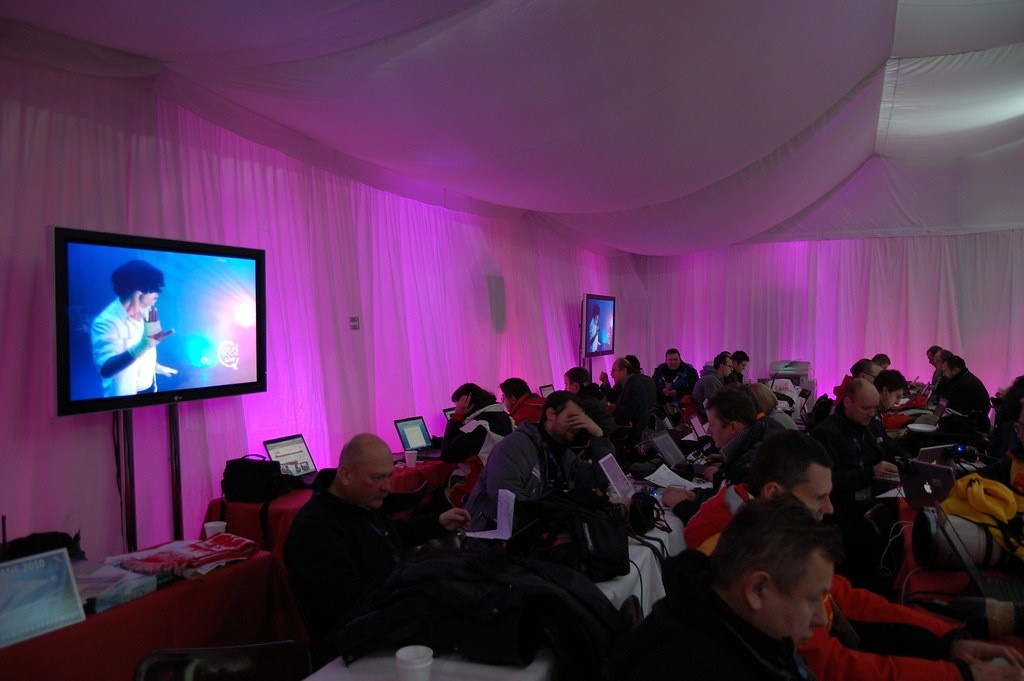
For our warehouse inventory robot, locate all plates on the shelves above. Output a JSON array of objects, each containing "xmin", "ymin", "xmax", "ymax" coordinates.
[{"xmin": 907, "ymin": 424, "xmax": 937, "ymax": 432}]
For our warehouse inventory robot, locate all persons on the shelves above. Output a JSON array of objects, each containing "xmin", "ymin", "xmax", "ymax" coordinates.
[
  {"xmin": 89, "ymin": 260, "xmax": 179, "ymax": 399},
  {"xmin": 442, "ymin": 342, "xmax": 1023, "ymax": 681},
  {"xmin": 584, "ymin": 304, "xmax": 607, "ymax": 351},
  {"xmin": 281, "ymin": 433, "xmax": 474, "ymax": 671}
]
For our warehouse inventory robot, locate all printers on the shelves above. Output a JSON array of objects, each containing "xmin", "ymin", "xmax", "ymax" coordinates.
[{"xmin": 769, "ymin": 361, "xmax": 810, "ymax": 378}]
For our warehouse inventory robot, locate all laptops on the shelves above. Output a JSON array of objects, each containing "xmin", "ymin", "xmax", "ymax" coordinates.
[
  {"xmin": 264, "ymin": 433, "xmax": 318, "ymax": 485},
  {"xmin": 895, "ymin": 376, "xmax": 959, "ymax": 510},
  {"xmin": 599, "ymin": 452, "xmax": 671, "ymax": 511},
  {"xmin": 648, "ymin": 430, "xmax": 708, "ymax": 478},
  {"xmin": 703, "ymin": 398, "xmax": 708, "ymax": 408},
  {"xmin": 539, "ymin": 384, "xmax": 555, "ymax": 398},
  {"xmin": 770, "ymin": 372, "xmax": 778, "ymax": 389},
  {"xmin": 796, "ymin": 389, "xmax": 811, "ymax": 413},
  {"xmin": 933, "ymin": 499, "xmax": 1024, "ymax": 604},
  {"xmin": 689, "ymin": 413, "xmax": 707, "ymax": 439},
  {"xmin": 394, "ymin": 415, "xmax": 441, "ymax": 461},
  {"xmin": 443, "ymin": 408, "xmax": 455, "ymax": 421}
]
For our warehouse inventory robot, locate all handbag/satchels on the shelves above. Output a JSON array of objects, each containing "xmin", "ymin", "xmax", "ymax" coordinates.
[{"xmin": 221, "ymin": 454, "xmax": 287, "ymax": 503}]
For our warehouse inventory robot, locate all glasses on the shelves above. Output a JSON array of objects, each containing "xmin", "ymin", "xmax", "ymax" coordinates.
[
  {"xmin": 721, "ymin": 362, "xmax": 733, "ymax": 369},
  {"xmin": 610, "ymin": 369, "xmax": 621, "ymax": 374}
]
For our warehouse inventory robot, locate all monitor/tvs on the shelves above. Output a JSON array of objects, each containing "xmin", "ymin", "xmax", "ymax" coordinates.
[
  {"xmin": 581, "ymin": 293, "xmax": 615, "ymax": 358},
  {"xmin": 44, "ymin": 226, "xmax": 267, "ymax": 416}
]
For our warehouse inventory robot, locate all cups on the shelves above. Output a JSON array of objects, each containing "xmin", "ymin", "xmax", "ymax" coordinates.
[
  {"xmin": 395, "ymin": 646, "xmax": 433, "ymax": 681},
  {"xmin": 405, "ymin": 451, "xmax": 417, "ymax": 467},
  {"xmin": 205, "ymin": 521, "xmax": 227, "ymax": 538}
]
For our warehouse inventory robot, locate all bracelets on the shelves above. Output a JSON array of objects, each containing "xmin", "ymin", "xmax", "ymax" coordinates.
[{"xmin": 591, "ymin": 426, "xmax": 600, "ymax": 435}]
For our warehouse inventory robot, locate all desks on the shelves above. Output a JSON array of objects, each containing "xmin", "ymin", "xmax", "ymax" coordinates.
[{"xmin": 1, "ymin": 381, "xmax": 1024, "ymax": 681}]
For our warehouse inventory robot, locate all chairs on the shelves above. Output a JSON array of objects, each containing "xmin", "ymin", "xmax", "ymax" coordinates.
[
  {"xmin": 800, "ymin": 407, "xmax": 809, "ymax": 426},
  {"xmin": 798, "ymin": 389, "xmax": 812, "ymax": 414},
  {"xmin": 865, "ymin": 503, "xmax": 902, "ymax": 563},
  {"xmin": 384, "ymin": 481, "xmax": 428, "ymax": 520},
  {"xmin": 132, "ymin": 639, "xmax": 312, "ymax": 680},
  {"xmin": 610, "ymin": 421, "xmax": 632, "ymax": 455},
  {"xmin": 665, "ymin": 402, "xmax": 686, "ymax": 427},
  {"xmin": 569, "ymin": 440, "xmax": 591, "ymax": 455}
]
